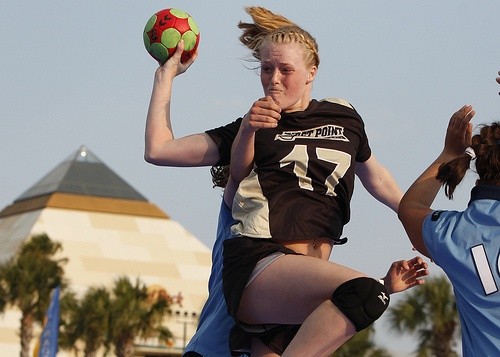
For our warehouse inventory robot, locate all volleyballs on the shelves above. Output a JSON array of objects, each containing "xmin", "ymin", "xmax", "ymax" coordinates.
[{"xmin": 143, "ymin": 7, "xmax": 201, "ymax": 63}]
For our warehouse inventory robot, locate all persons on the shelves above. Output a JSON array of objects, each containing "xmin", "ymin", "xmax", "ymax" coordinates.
[
  {"xmin": 140, "ymin": 3, "xmax": 411, "ymax": 357},
  {"xmin": 178, "ymin": 91, "xmax": 429, "ymax": 357},
  {"xmin": 396, "ymin": 69, "xmax": 500, "ymax": 357}
]
